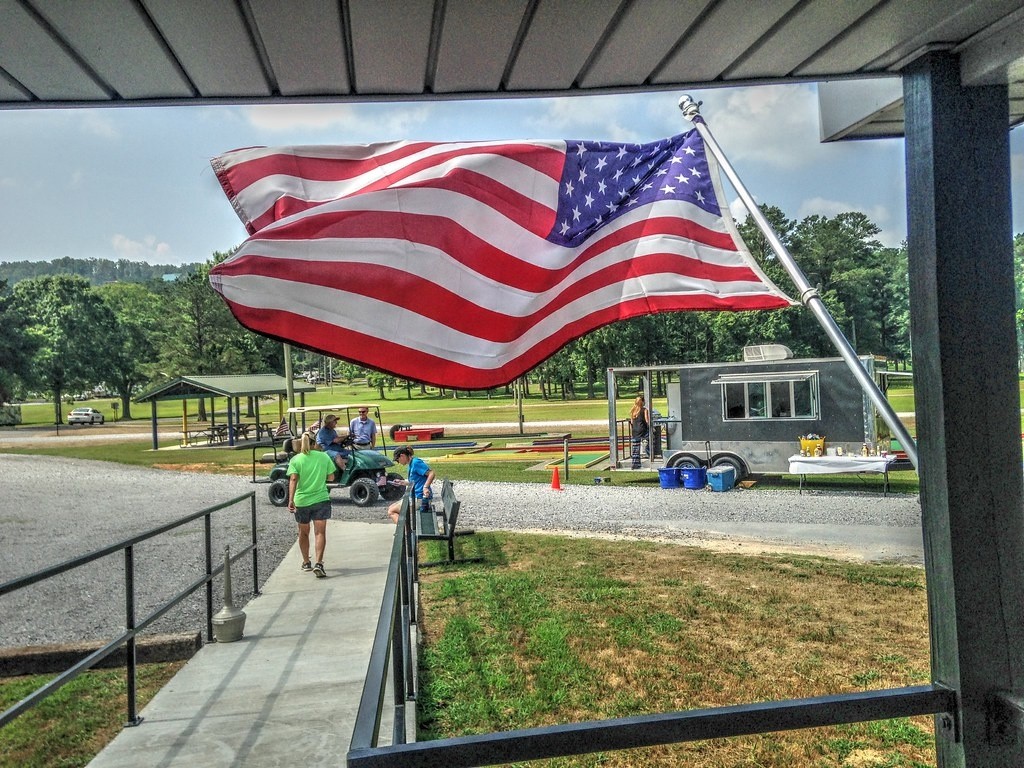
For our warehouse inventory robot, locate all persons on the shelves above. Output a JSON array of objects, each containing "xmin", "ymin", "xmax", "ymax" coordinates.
[
  {"xmin": 286, "ymin": 430, "xmax": 336, "ymax": 577},
  {"xmin": 347, "ymin": 407, "xmax": 377, "ymax": 451},
  {"xmin": 315, "ymin": 414, "xmax": 354, "ymax": 472},
  {"xmin": 387, "ymin": 446, "xmax": 435, "ymax": 536},
  {"xmin": 629, "ymin": 395, "xmax": 656, "ymax": 469}
]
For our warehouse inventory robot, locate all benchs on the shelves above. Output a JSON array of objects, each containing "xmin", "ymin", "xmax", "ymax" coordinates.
[
  {"xmin": 175, "ymin": 436, "xmax": 211, "ymax": 441},
  {"xmin": 292, "ymin": 439, "xmax": 303, "ymax": 453},
  {"xmin": 405, "ymin": 479, "xmax": 485, "ymax": 568},
  {"xmin": 246, "ymin": 427, "xmax": 276, "ymax": 431},
  {"xmin": 205, "ymin": 433, "xmax": 227, "ymax": 437},
  {"xmin": 263, "ymin": 435, "xmax": 301, "ymax": 460}
]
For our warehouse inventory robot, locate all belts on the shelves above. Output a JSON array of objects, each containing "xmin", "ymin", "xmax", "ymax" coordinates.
[{"xmin": 354, "ymin": 442, "xmax": 370, "ymax": 446}]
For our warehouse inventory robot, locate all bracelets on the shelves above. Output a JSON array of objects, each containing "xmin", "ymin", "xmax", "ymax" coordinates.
[{"xmin": 423, "ymin": 485, "xmax": 428, "ymax": 488}]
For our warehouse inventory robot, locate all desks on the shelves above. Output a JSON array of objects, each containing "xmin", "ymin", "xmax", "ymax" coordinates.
[
  {"xmin": 175, "ymin": 422, "xmax": 275, "ymax": 449},
  {"xmin": 788, "ymin": 454, "xmax": 897, "ymax": 497}
]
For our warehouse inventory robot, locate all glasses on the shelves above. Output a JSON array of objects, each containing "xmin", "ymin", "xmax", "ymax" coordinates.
[{"xmin": 359, "ymin": 411, "xmax": 367, "ymax": 413}]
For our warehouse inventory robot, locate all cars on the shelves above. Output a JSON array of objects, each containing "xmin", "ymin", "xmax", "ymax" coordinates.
[{"xmin": 68, "ymin": 408, "xmax": 105, "ymax": 426}]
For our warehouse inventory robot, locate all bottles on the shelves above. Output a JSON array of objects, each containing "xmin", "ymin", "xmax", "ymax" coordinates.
[
  {"xmin": 806, "ymin": 447, "xmax": 810, "ymax": 457},
  {"xmin": 817, "ymin": 443, "xmax": 822, "ymax": 456},
  {"xmin": 861, "ymin": 443, "xmax": 888, "ymax": 458},
  {"xmin": 837, "ymin": 446, "xmax": 843, "ymax": 456},
  {"xmin": 801, "ymin": 449, "xmax": 805, "ymax": 457}
]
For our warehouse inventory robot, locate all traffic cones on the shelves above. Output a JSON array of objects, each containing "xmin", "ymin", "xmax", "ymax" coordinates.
[{"xmin": 552, "ymin": 467, "xmax": 565, "ymax": 492}]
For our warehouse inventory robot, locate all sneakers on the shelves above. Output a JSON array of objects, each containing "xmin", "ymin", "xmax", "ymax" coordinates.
[
  {"xmin": 312, "ymin": 562, "xmax": 326, "ymax": 577},
  {"xmin": 301, "ymin": 561, "xmax": 312, "ymax": 571}
]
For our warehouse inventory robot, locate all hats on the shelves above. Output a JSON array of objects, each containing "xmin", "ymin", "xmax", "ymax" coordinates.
[
  {"xmin": 324, "ymin": 415, "xmax": 340, "ymax": 423},
  {"xmin": 393, "ymin": 447, "xmax": 403, "ymax": 460}
]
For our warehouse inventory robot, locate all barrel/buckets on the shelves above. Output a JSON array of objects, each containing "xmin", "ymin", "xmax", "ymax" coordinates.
[
  {"xmin": 657, "ymin": 468, "xmax": 682, "ymax": 489},
  {"xmin": 682, "ymin": 468, "xmax": 706, "ymax": 489}
]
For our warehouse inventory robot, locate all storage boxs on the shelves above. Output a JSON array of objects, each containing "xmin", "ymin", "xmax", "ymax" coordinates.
[{"xmin": 707, "ymin": 466, "xmax": 736, "ymax": 492}]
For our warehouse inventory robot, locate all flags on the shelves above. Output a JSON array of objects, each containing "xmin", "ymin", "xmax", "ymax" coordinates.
[
  {"xmin": 274, "ymin": 418, "xmax": 289, "ymax": 436},
  {"xmin": 207, "ymin": 115, "xmax": 802, "ymax": 391},
  {"xmin": 308, "ymin": 417, "xmax": 323, "ymax": 430}
]
